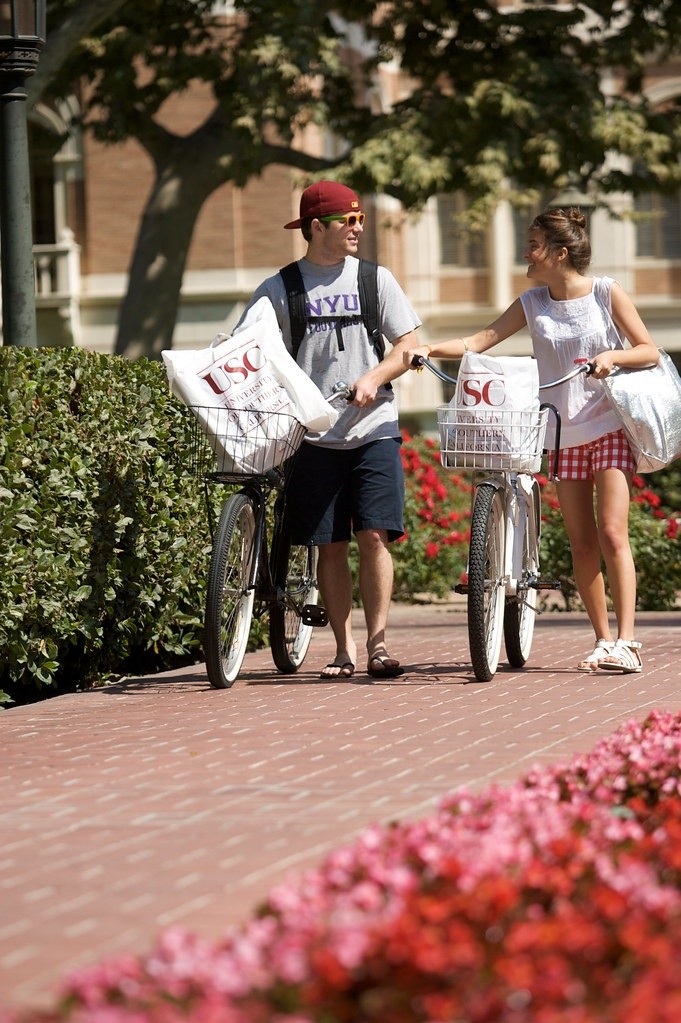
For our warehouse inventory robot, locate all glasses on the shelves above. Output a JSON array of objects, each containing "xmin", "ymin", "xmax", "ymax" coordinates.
[{"xmin": 319, "ymin": 213, "xmax": 365, "ymax": 228}]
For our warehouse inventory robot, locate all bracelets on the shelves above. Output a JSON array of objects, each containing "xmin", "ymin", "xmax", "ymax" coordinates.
[
  {"xmin": 461, "ymin": 337, "xmax": 468, "ymax": 351},
  {"xmin": 424, "ymin": 344, "xmax": 432, "ymax": 354}
]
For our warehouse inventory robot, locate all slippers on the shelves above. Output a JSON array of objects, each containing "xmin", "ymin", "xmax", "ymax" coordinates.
[
  {"xmin": 367, "ymin": 657, "xmax": 404, "ymax": 676},
  {"xmin": 321, "ymin": 663, "xmax": 354, "ymax": 679}
]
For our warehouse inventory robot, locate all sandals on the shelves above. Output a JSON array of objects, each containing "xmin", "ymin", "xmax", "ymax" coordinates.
[
  {"xmin": 598, "ymin": 638, "xmax": 643, "ymax": 672},
  {"xmin": 578, "ymin": 638, "xmax": 615, "ymax": 670}
]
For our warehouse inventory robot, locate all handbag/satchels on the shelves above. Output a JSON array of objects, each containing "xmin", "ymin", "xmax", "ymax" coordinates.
[
  {"xmin": 448, "ymin": 351, "xmax": 539, "ymax": 467},
  {"xmin": 602, "ymin": 347, "xmax": 681, "ymax": 474},
  {"xmin": 161, "ymin": 296, "xmax": 340, "ymax": 477}
]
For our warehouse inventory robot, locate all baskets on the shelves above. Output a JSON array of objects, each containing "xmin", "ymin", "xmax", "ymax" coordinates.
[
  {"xmin": 437, "ymin": 403, "xmax": 549, "ymax": 474},
  {"xmin": 187, "ymin": 405, "xmax": 307, "ymax": 490}
]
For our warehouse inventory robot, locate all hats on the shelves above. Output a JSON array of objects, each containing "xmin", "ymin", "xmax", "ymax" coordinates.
[{"xmin": 284, "ymin": 180, "xmax": 361, "ymax": 229}]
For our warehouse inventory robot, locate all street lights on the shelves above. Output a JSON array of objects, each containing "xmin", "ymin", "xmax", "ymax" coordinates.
[{"xmin": 0, "ymin": 0, "xmax": 45, "ymax": 350}]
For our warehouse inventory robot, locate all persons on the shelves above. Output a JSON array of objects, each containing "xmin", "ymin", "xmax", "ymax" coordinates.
[
  {"xmin": 403, "ymin": 205, "xmax": 660, "ymax": 672},
  {"xmin": 232, "ymin": 182, "xmax": 424, "ymax": 679}
]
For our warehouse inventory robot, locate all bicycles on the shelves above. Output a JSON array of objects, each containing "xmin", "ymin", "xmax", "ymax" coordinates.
[
  {"xmin": 413, "ymin": 350, "xmax": 595, "ymax": 683},
  {"xmin": 171, "ymin": 381, "xmax": 356, "ymax": 690}
]
